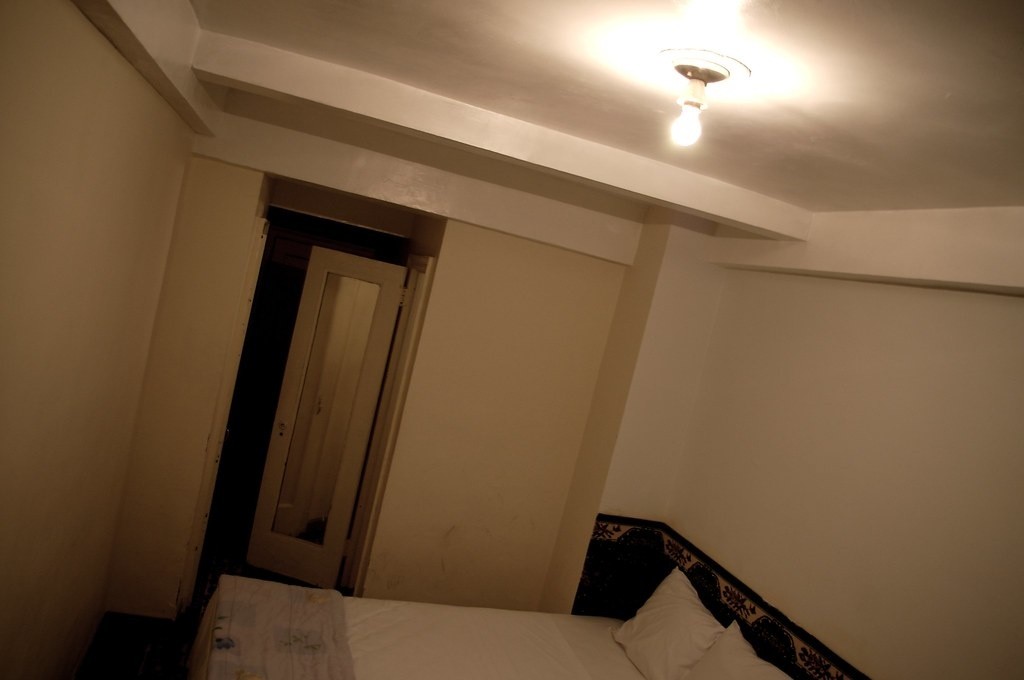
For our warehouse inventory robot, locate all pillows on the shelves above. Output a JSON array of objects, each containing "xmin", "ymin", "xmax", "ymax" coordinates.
[
  {"xmin": 613, "ymin": 564, "xmax": 725, "ymax": 680},
  {"xmin": 682, "ymin": 620, "xmax": 791, "ymax": 679}
]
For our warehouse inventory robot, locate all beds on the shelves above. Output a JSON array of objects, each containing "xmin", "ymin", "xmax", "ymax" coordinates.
[{"xmin": 191, "ymin": 574, "xmax": 644, "ymax": 680}]
